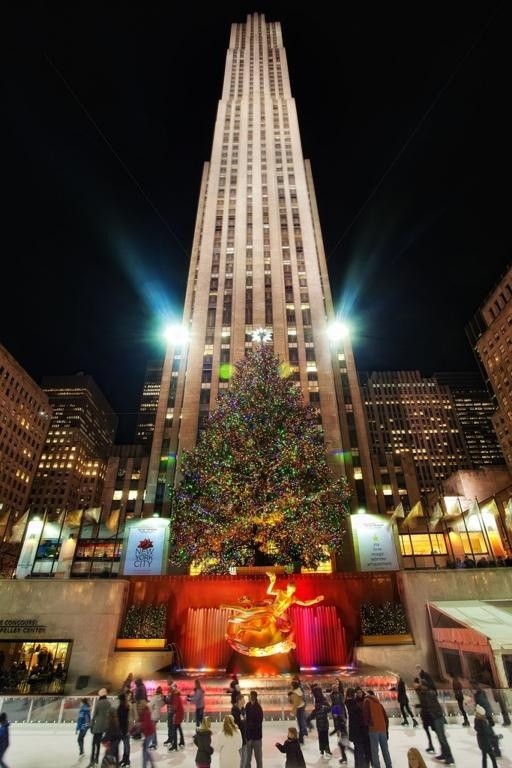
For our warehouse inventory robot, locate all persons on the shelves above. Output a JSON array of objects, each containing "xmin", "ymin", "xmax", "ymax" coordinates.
[
  {"xmin": 276, "ymin": 666, "xmax": 512, "ymax": 768},
  {"xmin": 76, "ymin": 662, "xmax": 262, "ymax": 767},
  {"xmin": 0, "ymin": 710, "xmax": 9, "ymax": 765},
  {"xmin": 220, "ymin": 569, "xmax": 326, "ymax": 623},
  {"xmin": 445, "ymin": 553, "xmax": 511, "ymax": 567},
  {"xmin": 8, "ymin": 658, "xmax": 64, "ymax": 689}
]
